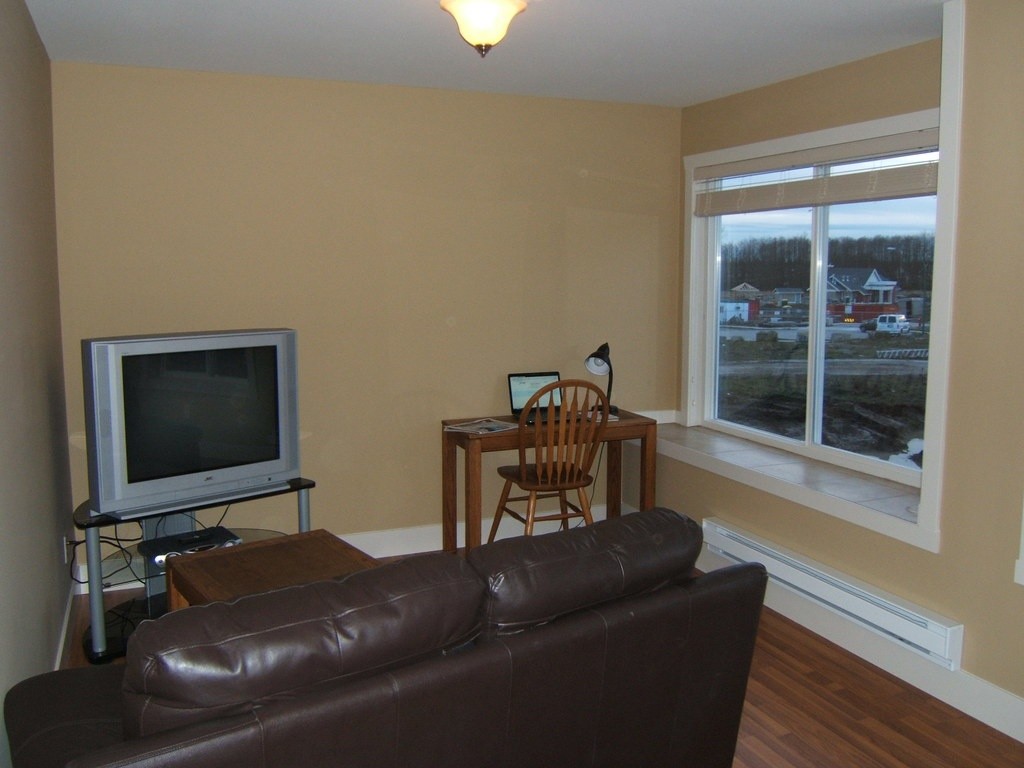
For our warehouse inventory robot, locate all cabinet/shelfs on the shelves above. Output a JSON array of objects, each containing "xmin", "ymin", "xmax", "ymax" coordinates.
[{"xmin": 73, "ymin": 475, "xmax": 316, "ymax": 668}]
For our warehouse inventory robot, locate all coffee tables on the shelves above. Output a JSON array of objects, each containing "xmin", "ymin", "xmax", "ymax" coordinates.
[{"xmin": 167, "ymin": 529, "xmax": 388, "ymax": 616}]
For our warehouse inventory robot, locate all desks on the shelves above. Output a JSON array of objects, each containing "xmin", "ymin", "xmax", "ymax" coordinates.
[{"xmin": 441, "ymin": 405, "xmax": 655, "ymax": 552}]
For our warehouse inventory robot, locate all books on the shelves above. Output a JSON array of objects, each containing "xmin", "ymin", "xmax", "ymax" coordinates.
[{"xmin": 577, "ymin": 410, "xmax": 620, "ymax": 424}]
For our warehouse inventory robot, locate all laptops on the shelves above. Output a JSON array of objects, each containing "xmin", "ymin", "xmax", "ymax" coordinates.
[{"xmin": 508, "ymin": 371, "xmax": 591, "ymax": 424}]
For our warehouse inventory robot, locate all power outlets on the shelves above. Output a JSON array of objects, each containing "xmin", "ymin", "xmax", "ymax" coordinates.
[{"xmin": 63, "ymin": 534, "xmax": 73, "ymax": 564}]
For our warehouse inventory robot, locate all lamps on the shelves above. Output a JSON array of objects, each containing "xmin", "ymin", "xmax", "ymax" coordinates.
[
  {"xmin": 584, "ymin": 342, "xmax": 619, "ymax": 413},
  {"xmin": 440, "ymin": 0, "xmax": 529, "ymax": 58}
]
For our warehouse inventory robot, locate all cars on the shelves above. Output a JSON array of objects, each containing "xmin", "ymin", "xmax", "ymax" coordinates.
[
  {"xmin": 859, "ymin": 319, "xmax": 877, "ymax": 332},
  {"xmin": 764, "ymin": 317, "xmax": 790, "ymax": 328}
]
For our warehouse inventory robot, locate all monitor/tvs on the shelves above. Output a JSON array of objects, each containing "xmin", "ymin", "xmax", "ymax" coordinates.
[{"xmin": 79, "ymin": 327, "xmax": 300, "ymax": 521}]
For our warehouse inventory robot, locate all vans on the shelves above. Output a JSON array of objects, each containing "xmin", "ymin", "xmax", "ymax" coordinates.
[{"xmin": 876, "ymin": 314, "xmax": 910, "ymax": 335}]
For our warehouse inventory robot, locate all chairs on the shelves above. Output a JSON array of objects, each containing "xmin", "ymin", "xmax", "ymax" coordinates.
[{"xmin": 486, "ymin": 379, "xmax": 610, "ymax": 545}]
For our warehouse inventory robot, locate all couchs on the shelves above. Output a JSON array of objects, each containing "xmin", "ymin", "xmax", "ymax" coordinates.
[{"xmin": 1, "ymin": 507, "xmax": 768, "ymax": 768}]
